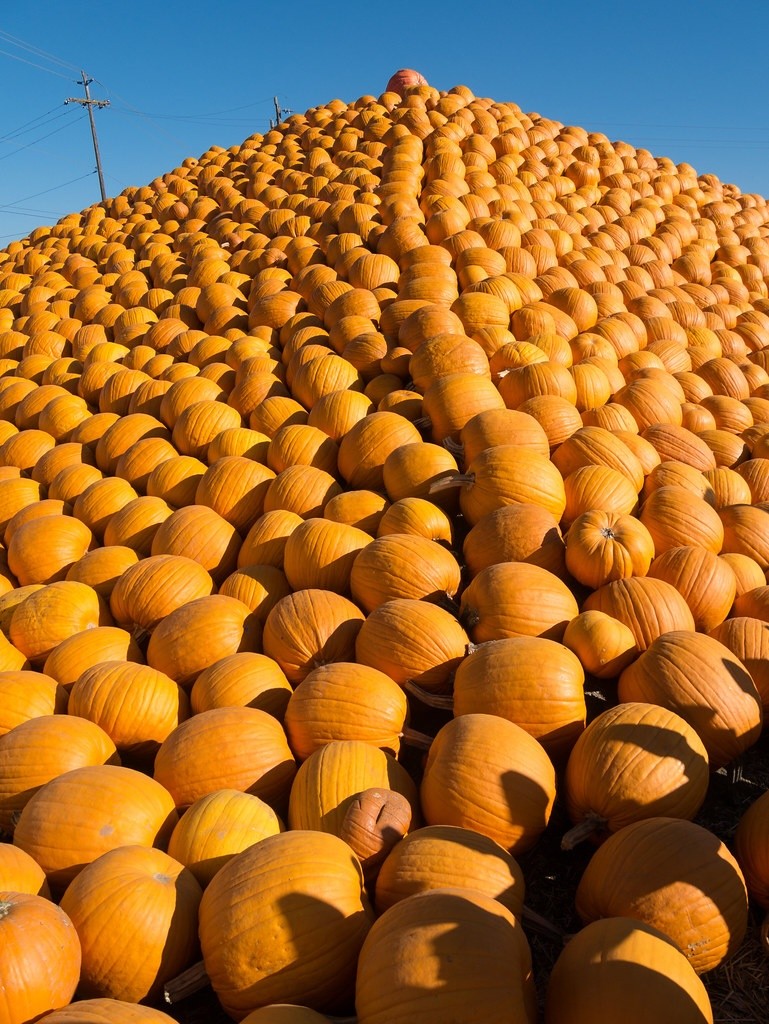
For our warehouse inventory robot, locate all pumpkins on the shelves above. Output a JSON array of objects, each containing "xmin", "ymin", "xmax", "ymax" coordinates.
[{"xmin": 0, "ymin": 68, "xmax": 769, "ymax": 1024}]
